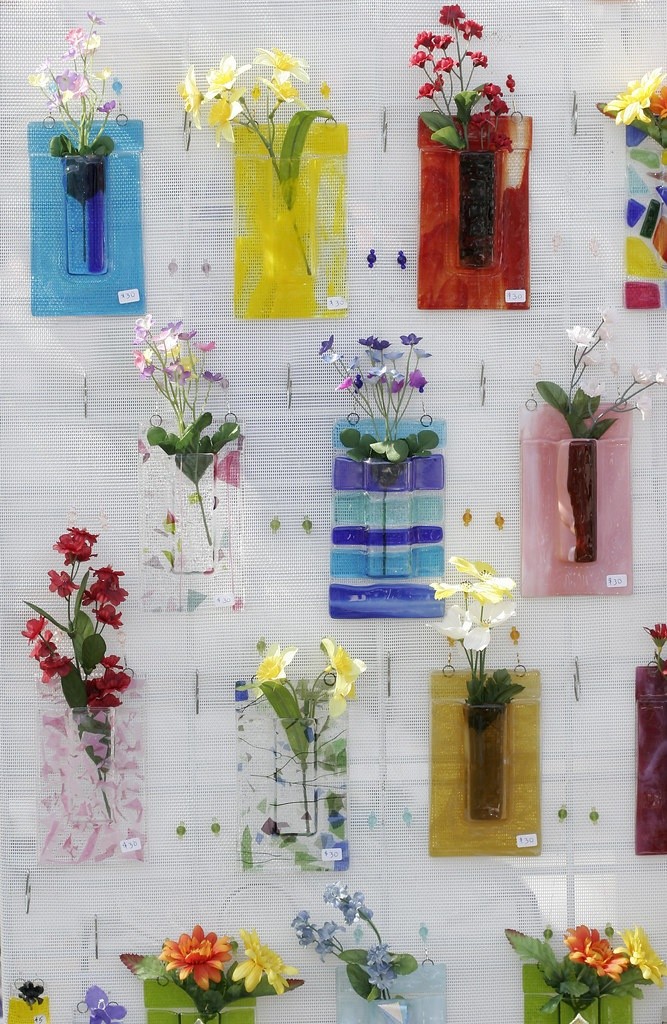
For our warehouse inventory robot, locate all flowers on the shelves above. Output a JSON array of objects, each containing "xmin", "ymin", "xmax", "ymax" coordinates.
[
  {"xmin": 535, "ymin": 306, "xmax": 666, "ymax": 439},
  {"xmin": 120, "ymin": 927, "xmax": 305, "ymax": 1023},
  {"xmin": 426, "ymin": 557, "xmax": 525, "ymax": 728},
  {"xmin": 21, "ymin": 528, "xmax": 131, "ymax": 822},
  {"xmin": 643, "ymin": 623, "xmax": 667, "ymax": 678},
  {"xmin": 505, "ymin": 924, "xmax": 667, "ymax": 1016},
  {"xmin": 414, "ymin": 4, "xmax": 520, "ymax": 152},
  {"xmin": 317, "ymin": 335, "xmax": 439, "ymax": 573},
  {"xmin": 597, "ymin": 66, "xmax": 667, "ymax": 149},
  {"xmin": 234, "ymin": 637, "xmax": 368, "ymax": 834},
  {"xmin": 131, "ymin": 315, "xmax": 243, "ymax": 545},
  {"xmin": 177, "ymin": 48, "xmax": 338, "ymax": 275},
  {"xmin": 293, "ymin": 882, "xmax": 417, "ymax": 1002},
  {"xmin": 30, "ymin": 15, "xmax": 123, "ymax": 261}
]
